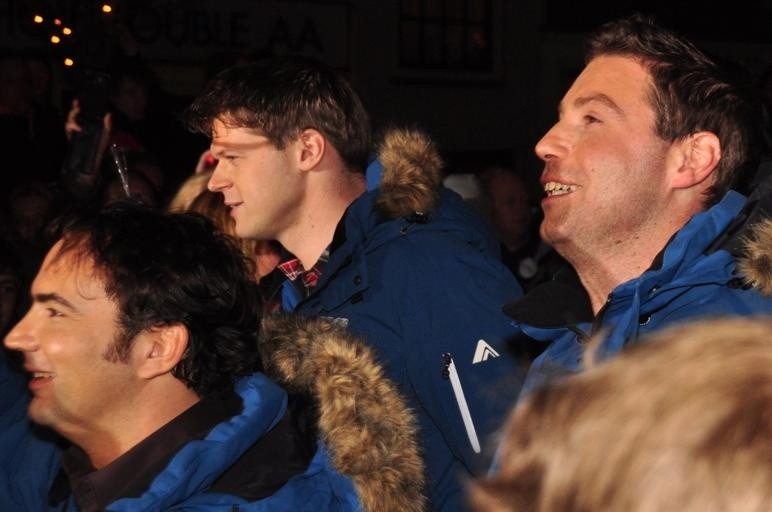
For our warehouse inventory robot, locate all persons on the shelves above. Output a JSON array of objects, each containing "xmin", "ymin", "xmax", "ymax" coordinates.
[
  {"xmin": 484, "ymin": 17, "xmax": 772, "ymax": 490},
  {"xmin": 187, "ymin": 50, "xmax": 555, "ymax": 512},
  {"xmin": 457, "ymin": 313, "xmax": 772, "ymax": 512},
  {"xmin": 1, "ymin": 202, "xmax": 427, "ymax": 512},
  {"xmin": 2, "ymin": 3, "xmax": 281, "ymax": 414},
  {"xmin": 441, "ymin": 165, "xmax": 565, "ymax": 295},
  {"xmin": 709, "ymin": 56, "xmax": 772, "ymax": 215}
]
